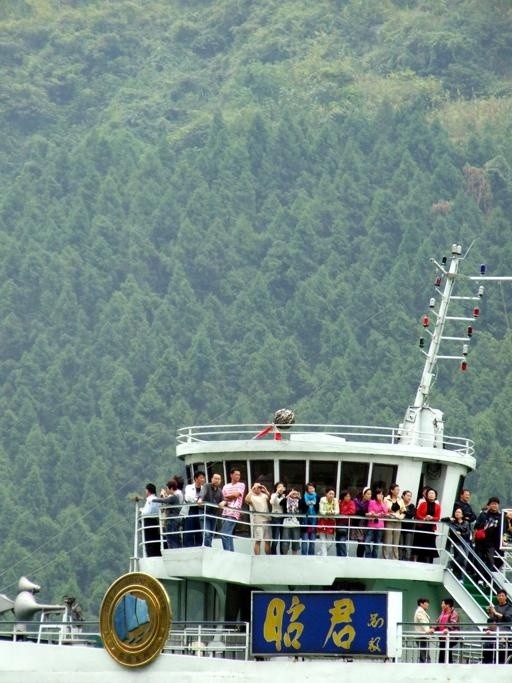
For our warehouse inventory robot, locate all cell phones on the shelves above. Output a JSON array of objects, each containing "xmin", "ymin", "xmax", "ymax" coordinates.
[{"xmin": 281, "ymin": 487, "xmax": 287, "ymax": 491}]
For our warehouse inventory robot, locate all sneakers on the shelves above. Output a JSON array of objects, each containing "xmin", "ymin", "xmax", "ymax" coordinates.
[{"xmin": 477, "ymin": 579, "xmax": 484, "ymax": 586}]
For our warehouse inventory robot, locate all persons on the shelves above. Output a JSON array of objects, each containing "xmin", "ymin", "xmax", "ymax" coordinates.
[
  {"xmin": 483, "ymin": 618, "xmax": 511, "ymax": 663},
  {"xmin": 431, "ymin": 599, "xmax": 459, "ymax": 663},
  {"xmin": 414, "ymin": 599, "xmax": 431, "ymax": 664},
  {"xmin": 489, "ymin": 589, "xmax": 512, "ymax": 631}
]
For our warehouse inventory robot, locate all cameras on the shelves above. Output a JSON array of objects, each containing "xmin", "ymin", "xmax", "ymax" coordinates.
[
  {"xmin": 258, "ymin": 485, "xmax": 263, "ymax": 489},
  {"xmin": 293, "ymin": 492, "xmax": 300, "ymax": 497}
]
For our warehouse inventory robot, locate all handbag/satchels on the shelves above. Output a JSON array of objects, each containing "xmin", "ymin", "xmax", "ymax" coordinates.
[
  {"xmin": 317, "ymin": 519, "xmax": 336, "ymax": 533},
  {"xmin": 476, "ymin": 529, "xmax": 486, "ymax": 539}
]
